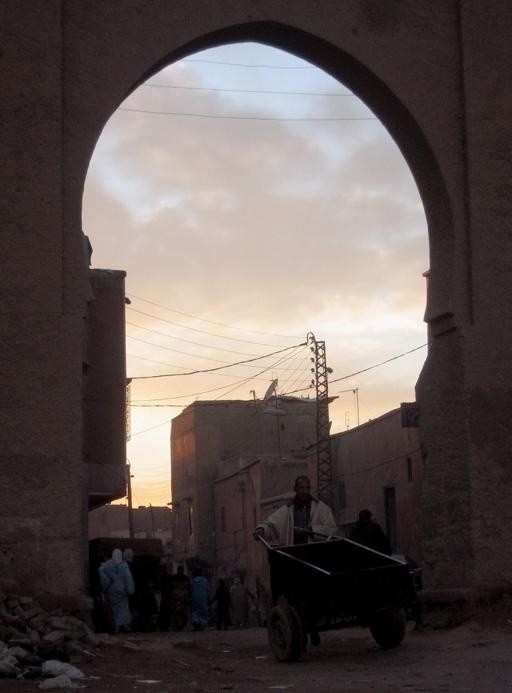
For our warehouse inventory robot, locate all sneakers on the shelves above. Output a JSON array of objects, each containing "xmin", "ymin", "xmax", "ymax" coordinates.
[{"xmin": 307, "ymin": 631, "xmax": 322, "ymax": 648}]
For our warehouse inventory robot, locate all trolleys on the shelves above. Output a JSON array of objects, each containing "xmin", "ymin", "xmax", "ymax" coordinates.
[{"xmin": 256, "ymin": 526, "xmax": 411, "ymax": 666}]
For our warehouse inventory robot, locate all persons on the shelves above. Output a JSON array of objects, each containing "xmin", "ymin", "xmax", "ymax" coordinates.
[
  {"xmin": 252, "ymin": 475, "xmax": 338, "ymax": 647},
  {"xmin": 99, "ymin": 546, "xmax": 267, "ymax": 634},
  {"xmin": 349, "ymin": 509, "xmax": 392, "ymax": 556}
]
